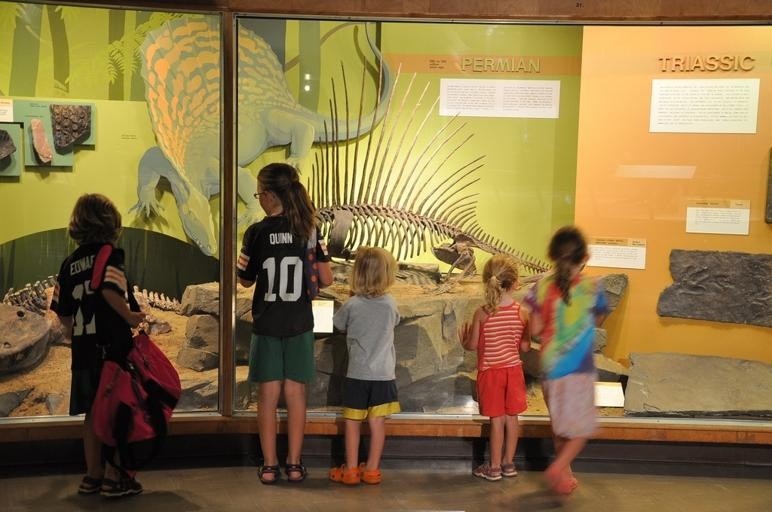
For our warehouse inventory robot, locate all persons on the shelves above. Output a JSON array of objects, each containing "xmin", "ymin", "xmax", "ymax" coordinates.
[
  {"xmin": 456, "ymin": 252, "xmax": 535, "ymax": 481},
  {"xmin": 236, "ymin": 162, "xmax": 332, "ymax": 486},
  {"xmin": 49, "ymin": 193, "xmax": 149, "ymax": 501},
  {"xmin": 517, "ymin": 227, "xmax": 612, "ymax": 495},
  {"xmin": 326, "ymin": 246, "xmax": 403, "ymax": 486}
]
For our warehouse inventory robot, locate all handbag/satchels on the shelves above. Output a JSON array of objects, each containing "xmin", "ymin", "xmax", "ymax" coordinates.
[{"xmin": 89, "ymin": 241, "xmax": 181, "ymax": 470}]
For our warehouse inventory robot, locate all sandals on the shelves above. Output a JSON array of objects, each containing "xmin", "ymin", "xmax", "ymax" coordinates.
[
  {"xmin": 285, "ymin": 461, "xmax": 307, "ymax": 482},
  {"xmin": 328, "ymin": 464, "xmax": 361, "ymax": 484},
  {"xmin": 358, "ymin": 463, "xmax": 382, "ymax": 483},
  {"xmin": 257, "ymin": 464, "xmax": 280, "ymax": 484}
]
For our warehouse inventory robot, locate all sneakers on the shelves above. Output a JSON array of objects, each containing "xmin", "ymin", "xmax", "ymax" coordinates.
[
  {"xmin": 78, "ymin": 476, "xmax": 101, "ymax": 493},
  {"xmin": 541, "ymin": 464, "xmax": 569, "ymax": 494},
  {"xmin": 473, "ymin": 459, "xmax": 502, "ymax": 481},
  {"xmin": 568, "ymin": 478, "xmax": 577, "ymax": 491},
  {"xmin": 499, "ymin": 463, "xmax": 518, "ymax": 476},
  {"xmin": 99, "ymin": 470, "xmax": 143, "ymax": 496}
]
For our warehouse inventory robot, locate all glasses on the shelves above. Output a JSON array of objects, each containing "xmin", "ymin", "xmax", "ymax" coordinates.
[{"xmin": 253, "ymin": 192, "xmax": 267, "ymax": 199}]
